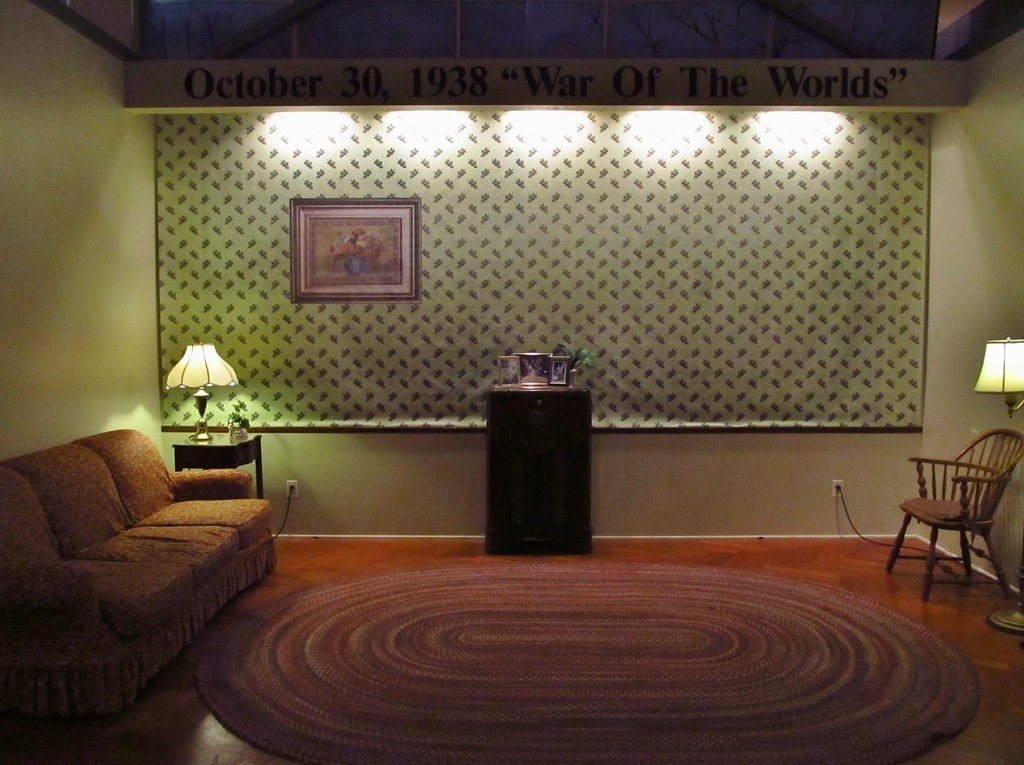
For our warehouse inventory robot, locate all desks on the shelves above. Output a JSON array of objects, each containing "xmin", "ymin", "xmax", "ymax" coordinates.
[{"xmin": 172, "ymin": 435, "xmax": 264, "ymax": 500}]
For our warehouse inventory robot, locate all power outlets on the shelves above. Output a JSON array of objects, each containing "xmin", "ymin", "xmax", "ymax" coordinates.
[
  {"xmin": 833, "ymin": 480, "xmax": 843, "ymax": 497},
  {"xmin": 287, "ymin": 480, "xmax": 298, "ymax": 498}
]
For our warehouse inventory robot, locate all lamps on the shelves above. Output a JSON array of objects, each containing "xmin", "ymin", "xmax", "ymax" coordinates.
[
  {"xmin": 975, "ymin": 337, "xmax": 1024, "ymax": 634},
  {"xmin": 166, "ymin": 343, "xmax": 239, "ymax": 440}
]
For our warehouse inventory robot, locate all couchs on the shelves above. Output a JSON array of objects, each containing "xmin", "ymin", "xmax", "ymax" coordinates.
[{"xmin": 0, "ymin": 430, "xmax": 278, "ymax": 722}]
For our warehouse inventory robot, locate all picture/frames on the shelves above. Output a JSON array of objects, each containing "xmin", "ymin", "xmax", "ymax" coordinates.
[
  {"xmin": 548, "ymin": 354, "xmax": 570, "ymax": 386},
  {"xmin": 497, "ymin": 356, "xmax": 521, "ymax": 387},
  {"xmin": 513, "ymin": 353, "xmax": 552, "ymax": 387},
  {"xmin": 289, "ymin": 197, "xmax": 422, "ymax": 303}
]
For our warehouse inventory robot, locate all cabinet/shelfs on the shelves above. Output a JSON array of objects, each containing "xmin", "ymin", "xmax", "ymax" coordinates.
[{"xmin": 483, "ymin": 385, "xmax": 593, "ymax": 556}]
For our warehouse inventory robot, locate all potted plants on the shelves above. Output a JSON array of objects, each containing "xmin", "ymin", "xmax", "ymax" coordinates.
[{"xmin": 552, "ymin": 344, "xmax": 596, "ymax": 387}]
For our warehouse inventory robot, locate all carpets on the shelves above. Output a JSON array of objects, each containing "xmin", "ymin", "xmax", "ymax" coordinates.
[{"xmin": 193, "ymin": 565, "xmax": 985, "ymax": 765}]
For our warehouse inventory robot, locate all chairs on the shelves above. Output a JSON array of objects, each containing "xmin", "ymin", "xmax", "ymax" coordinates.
[{"xmin": 886, "ymin": 429, "xmax": 1024, "ymax": 603}]
